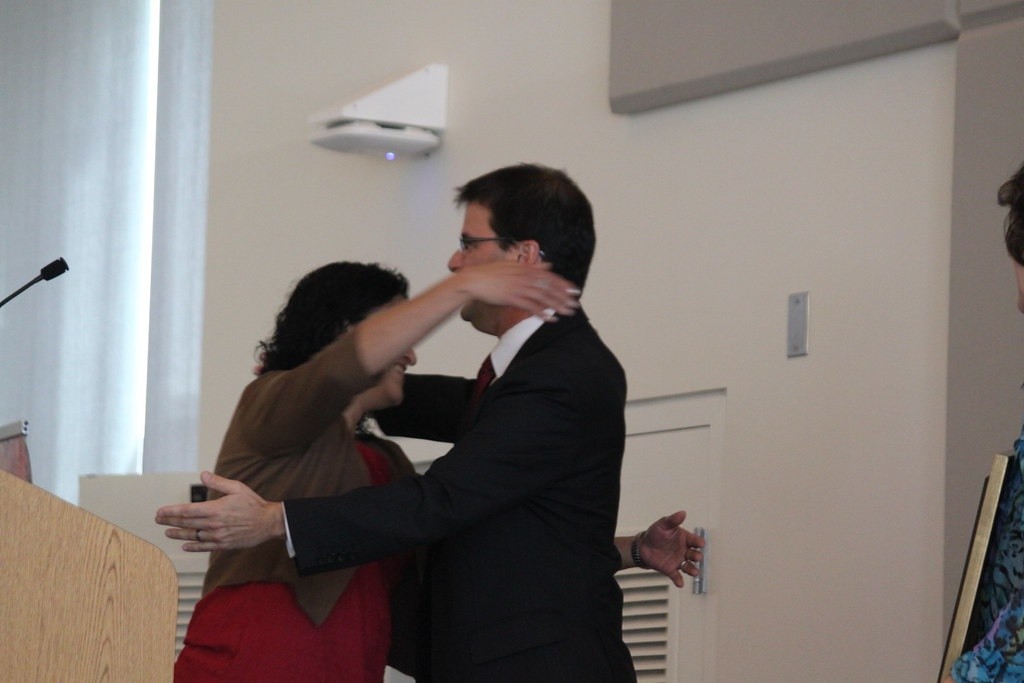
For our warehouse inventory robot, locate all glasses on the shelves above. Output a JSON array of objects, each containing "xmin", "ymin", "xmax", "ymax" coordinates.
[{"xmin": 459, "ymin": 234, "xmax": 546, "ymax": 261}]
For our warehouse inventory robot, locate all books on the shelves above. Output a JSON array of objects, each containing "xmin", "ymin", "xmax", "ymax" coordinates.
[{"xmin": 936, "ymin": 449, "xmax": 1016, "ymax": 683}]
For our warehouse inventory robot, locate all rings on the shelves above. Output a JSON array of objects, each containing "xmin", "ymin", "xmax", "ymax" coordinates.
[
  {"xmin": 195, "ymin": 529, "xmax": 201, "ymax": 541},
  {"xmin": 681, "ymin": 560, "xmax": 687, "ymax": 565}
]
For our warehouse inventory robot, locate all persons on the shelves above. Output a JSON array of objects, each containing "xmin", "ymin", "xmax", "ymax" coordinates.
[
  {"xmin": 155, "ymin": 161, "xmax": 640, "ymax": 683},
  {"xmin": 937, "ymin": 160, "xmax": 1023, "ymax": 683},
  {"xmin": 171, "ymin": 259, "xmax": 705, "ymax": 683}
]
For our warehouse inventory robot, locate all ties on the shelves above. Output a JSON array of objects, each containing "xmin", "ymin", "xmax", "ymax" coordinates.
[{"xmin": 467, "ymin": 353, "xmax": 495, "ymax": 428}]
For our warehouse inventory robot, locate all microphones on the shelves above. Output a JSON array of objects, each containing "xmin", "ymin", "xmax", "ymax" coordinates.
[{"xmin": 0, "ymin": 257, "xmax": 69, "ymax": 308}]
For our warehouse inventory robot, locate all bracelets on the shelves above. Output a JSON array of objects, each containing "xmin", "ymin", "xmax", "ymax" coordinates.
[{"xmin": 631, "ymin": 530, "xmax": 650, "ymax": 569}]
